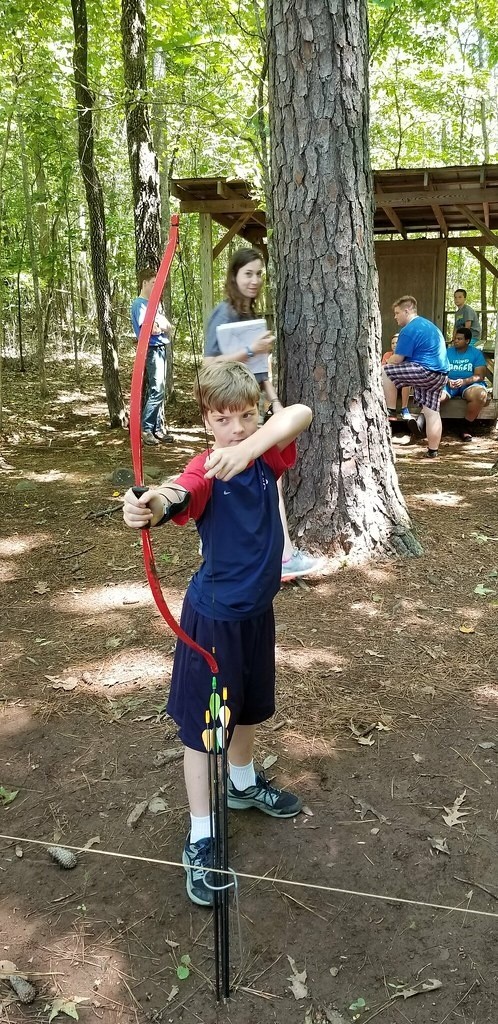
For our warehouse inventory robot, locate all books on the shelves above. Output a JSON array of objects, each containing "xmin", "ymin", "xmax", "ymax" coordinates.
[{"xmin": 216, "ymin": 319, "xmax": 270, "ymax": 373}]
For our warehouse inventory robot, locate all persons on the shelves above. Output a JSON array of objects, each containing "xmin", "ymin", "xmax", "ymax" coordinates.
[
  {"xmin": 204, "ymin": 247, "xmax": 284, "ymax": 413},
  {"xmin": 383, "ymin": 333, "xmax": 411, "ymax": 420},
  {"xmin": 453, "ymin": 289, "xmax": 479, "ymax": 346},
  {"xmin": 132, "ymin": 269, "xmax": 173, "ymax": 445},
  {"xmin": 123, "ymin": 359, "xmax": 313, "ymax": 907},
  {"xmin": 382, "ymin": 295, "xmax": 450, "ymax": 459},
  {"xmin": 409, "ymin": 328, "xmax": 487, "ymax": 441}
]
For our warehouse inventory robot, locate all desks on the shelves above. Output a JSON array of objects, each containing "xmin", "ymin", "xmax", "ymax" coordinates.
[{"xmin": 472, "ymin": 339, "xmax": 495, "ymax": 382}]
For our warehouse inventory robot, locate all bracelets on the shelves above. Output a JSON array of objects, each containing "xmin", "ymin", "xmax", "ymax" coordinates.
[
  {"xmin": 386, "ymin": 362, "xmax": 387, "ymax": 364},
  {"xmin": 156, "ymin": 322, "xmax": 159, "ymax": 327},
  {"xmin": 246, "ymin": 347, "xmax": 254, "ymax": 358},
  {"xmin": 270, "ymin": 398, "xmax": 279, "ymax": 403}
]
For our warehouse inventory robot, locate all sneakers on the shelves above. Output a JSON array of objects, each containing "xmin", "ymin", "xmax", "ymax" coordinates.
[
  {"xmin": 141, "ymin": 429, "xmax": 159, "ymax": 445},
  {"xmin": 226, "ymin": 770, "xmax": 302, "ymax": 818},
  {"xmin": 181, "ymin": 829, "xmax": 223, "ymax": 906},
  {"xmin": 153, "ymin": 429, "xmax": 173, "ymax": 443}
]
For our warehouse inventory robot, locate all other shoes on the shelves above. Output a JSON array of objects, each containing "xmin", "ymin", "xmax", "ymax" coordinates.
[
  {"xmin": 387, "ymin": 409, "xmax": 397, "ymax": 417},
  {"xmin": 400, "ymin": 407, "xmax": 413, "ymax": 420},
  {"xmin": 458, "ymin": 430, "xmax": 472, "ymax": 442},
  {"xmin": 411, "ymin": 447, "xmax": 439, "ymax": 458},
  {"xmin": 407, "ymin": 418, "xmax": 422, "ymax": 436}
]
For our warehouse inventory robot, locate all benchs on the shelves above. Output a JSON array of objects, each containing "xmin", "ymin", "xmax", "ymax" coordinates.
[{"xmin": 440, "ymin": 396, "xmax": 498, "ymax": 419}]
